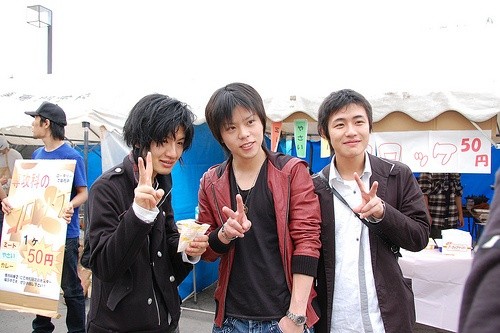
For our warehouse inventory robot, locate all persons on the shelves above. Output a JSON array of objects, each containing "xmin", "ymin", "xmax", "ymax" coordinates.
[
  {"xmin": 80, "ymin": 93, "xmax": 209, "ymax": 333},
  {"xmin": 1, "ymin": 101, "xmax": 88, "ymax": 333},
  {"xmin": 417, "ymin": 172, "xmax": 464, "ymax": 239},
  {"xmin": 0, "ymin": 132, "xmax": 24, "ymax": 247},
  {"xmin": 196, "ymin": 82, "xmax": 322, "ymax": 333},
  {"xmin": 311, "ymin": 89, "xmax": 430, "ymax": 333}
]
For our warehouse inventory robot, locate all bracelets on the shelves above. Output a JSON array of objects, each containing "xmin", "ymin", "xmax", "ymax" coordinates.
[{"xmin": 222, "ymin": 222, "xmax": 238, "ymax": 240}]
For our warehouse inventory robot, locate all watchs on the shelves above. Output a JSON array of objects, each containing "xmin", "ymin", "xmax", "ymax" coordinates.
[{"xmin": 286, "ymin": 310, "xmax": 307, "ymax": 326}]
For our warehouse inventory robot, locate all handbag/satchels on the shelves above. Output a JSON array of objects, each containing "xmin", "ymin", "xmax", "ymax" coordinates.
[{"xmin": 404, "ymin": 278, "xmax": 416, "ymax": 320}]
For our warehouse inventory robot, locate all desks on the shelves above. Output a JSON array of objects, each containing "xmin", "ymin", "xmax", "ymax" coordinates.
[{"xmin": 398, "ymin": 238, "xmax": 473, "ymax": 333}]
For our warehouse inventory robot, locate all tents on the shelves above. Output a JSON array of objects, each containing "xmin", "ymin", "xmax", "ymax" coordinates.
[{"xmin": 0, "ymin": 87, "xmax": 500, "ymax": 304}]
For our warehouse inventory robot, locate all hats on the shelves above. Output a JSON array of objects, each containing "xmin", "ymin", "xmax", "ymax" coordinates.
[{"xmin": 25, "ymin": 101, "xmax": 67, "ymax": 125}]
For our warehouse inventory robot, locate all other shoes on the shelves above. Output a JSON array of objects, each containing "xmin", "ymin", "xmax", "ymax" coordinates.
[{"xmin": 32, "ymin": 330, "xmax": 53, "ymax": 333}]
